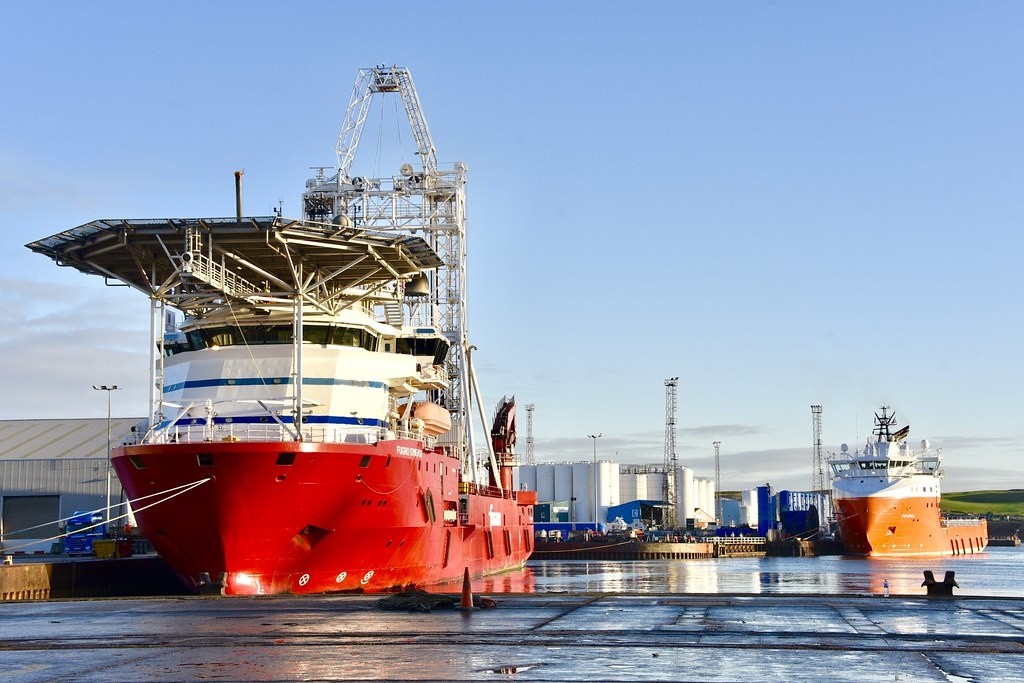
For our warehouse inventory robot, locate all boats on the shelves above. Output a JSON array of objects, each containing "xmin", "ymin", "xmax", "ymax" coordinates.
[
  {"xmin": 22, "ymin": 62, "xmax": 540, "ymax": 599},
  {"xmin": 823, "ymin": 399, "xmax": 989, "ymax": 556}
]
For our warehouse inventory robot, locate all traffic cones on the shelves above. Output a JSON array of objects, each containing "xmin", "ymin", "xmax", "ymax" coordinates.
[{"xmin": 452, "ymin": 567, "xmax": 482, "ymax": 612}]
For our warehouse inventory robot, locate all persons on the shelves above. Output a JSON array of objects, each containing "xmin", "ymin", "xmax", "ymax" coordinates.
[{"xmin": 124, "ymin": 522, "xmax": 131, "ymax": 534}]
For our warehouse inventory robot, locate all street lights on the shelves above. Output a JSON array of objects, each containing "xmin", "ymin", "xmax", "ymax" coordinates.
[
  {"xmin": 91, "ymin": 383, "xmax": 118, "ymax": 534},
  {"xmin": 587, "ymin": 432, "xmax": 603, "ymax": 534}
]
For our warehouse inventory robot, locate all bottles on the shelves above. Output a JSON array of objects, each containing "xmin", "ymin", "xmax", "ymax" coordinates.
[{"xmin": 883, "ymin": 579, "xmax": 889, "ymax": 597}]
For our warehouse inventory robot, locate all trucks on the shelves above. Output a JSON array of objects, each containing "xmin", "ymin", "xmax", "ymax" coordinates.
[{"xmin": 63, "ymin": 510, "xmax": 106, "ymax": 558}]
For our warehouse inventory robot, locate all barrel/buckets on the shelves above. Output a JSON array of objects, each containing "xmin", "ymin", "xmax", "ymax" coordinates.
[{"xmin": 119, "ymin": 540, "xmax": 132, "ymax": 557}]
